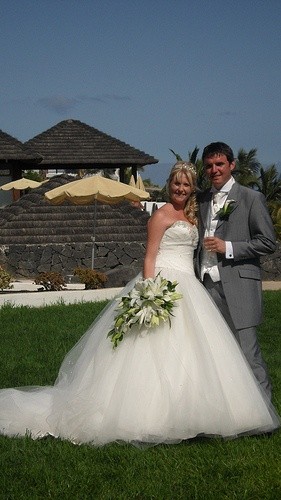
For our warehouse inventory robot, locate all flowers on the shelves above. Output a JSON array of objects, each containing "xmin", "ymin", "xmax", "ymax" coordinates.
[
  {"xmin": 107, "ymin": 270, "xmax": 183, "ymax": 351},
  {"xmin": 220, "ymin": 199, "xmax": 237, "ymax": 221}
]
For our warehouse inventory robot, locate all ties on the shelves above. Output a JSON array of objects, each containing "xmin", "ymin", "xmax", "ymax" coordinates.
[{"xmin": 211, "ymin": 191, "xmax": 227, "ymax": 213}]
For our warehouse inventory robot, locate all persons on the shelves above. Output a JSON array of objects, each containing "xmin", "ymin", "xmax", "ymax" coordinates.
[
  {"xmin": 143, "ymin": 161, "xmax": 200, "ymax": 444},
  {"xmin": 195, "ymin": 141, "xmax": 276, "ymax": 404}
]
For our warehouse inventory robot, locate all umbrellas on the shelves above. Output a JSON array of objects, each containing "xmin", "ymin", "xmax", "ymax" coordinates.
[
  {"xmin": 45, "ymin": 174, "xmax": 149, "ymax": 271},
  {"xmin": 129, "ymin": 174, "xmax": 145, "ymax": 192},
  {"xmin": 0, "ymin": 177, "xmax": 42, "ymax": 196}
]
